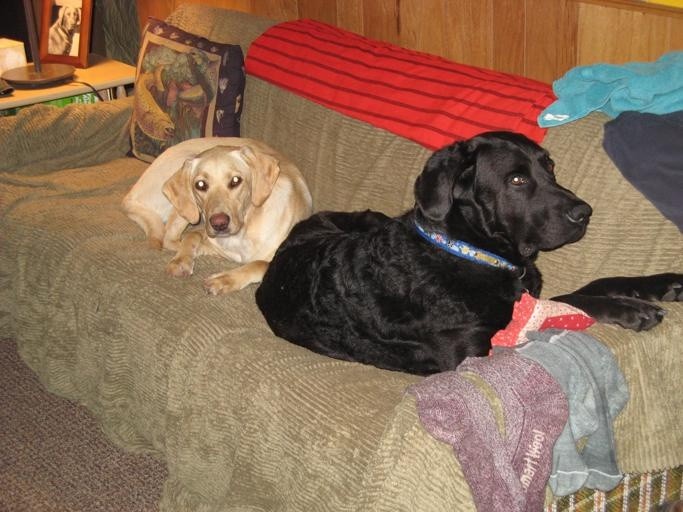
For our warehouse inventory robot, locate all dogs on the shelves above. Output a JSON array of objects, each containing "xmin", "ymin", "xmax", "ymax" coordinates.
[
  {"xmin": 48, "ymin": 6, "xmax": 82, "ymax": 56},
  {"xmin": 250, "ymin": 127, "xmax": 594, "ymax": 379},
  {"xmin": 118, "ymin": 133, "xmax": 316, "ymax": 300}
]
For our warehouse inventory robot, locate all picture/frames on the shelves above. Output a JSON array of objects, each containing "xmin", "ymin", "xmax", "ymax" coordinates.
[{"xmin": 38, "ymin": 0, "xmax": 94, "ymax": 69}]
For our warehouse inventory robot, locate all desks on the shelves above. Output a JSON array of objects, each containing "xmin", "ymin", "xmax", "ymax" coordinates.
[{"xmin": 0, "ymin": 52, "xmax": 136, "ymax": 110}]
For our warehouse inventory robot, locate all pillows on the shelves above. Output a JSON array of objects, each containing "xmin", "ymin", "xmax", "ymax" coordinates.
[{"xmin": 128, "ymin": 16, "xmax": 246, "ymax": 164}]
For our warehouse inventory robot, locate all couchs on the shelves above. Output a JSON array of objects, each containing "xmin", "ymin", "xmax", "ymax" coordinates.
[{"xmin": 0, "ymin": 4, "xmax": 683, "ymax": 512}]
